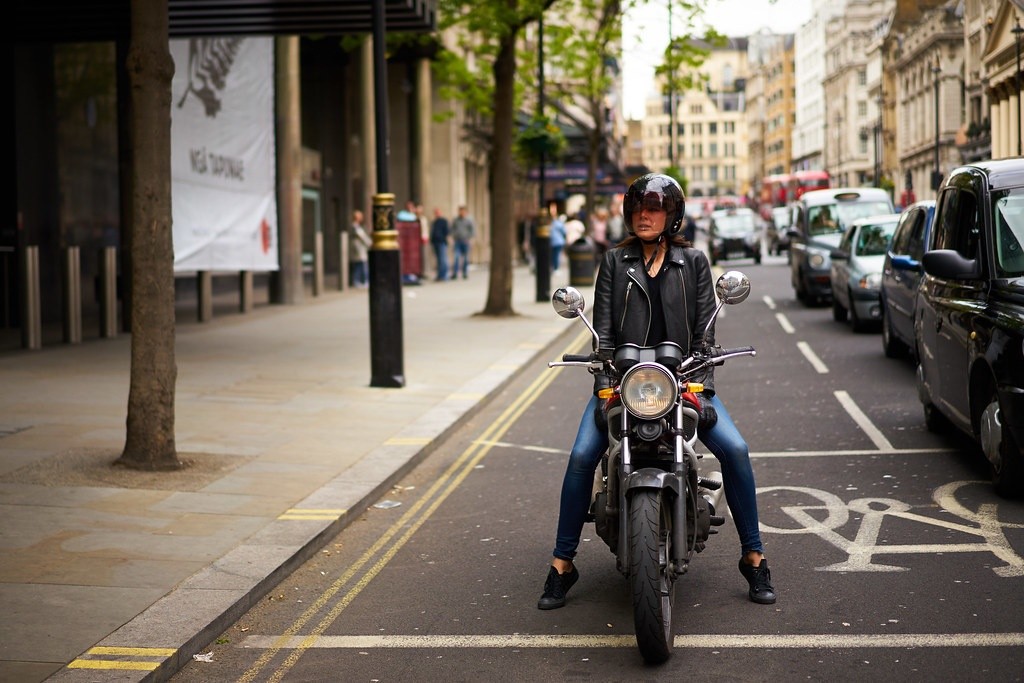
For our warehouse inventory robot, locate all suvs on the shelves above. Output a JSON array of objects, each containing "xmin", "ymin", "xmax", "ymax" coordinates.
[{"xmin": 911, "ymin": 155, "xmax": 1024, "ymax": 502}]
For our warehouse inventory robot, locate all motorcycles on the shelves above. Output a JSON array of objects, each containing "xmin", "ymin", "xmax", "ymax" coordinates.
[{"xmin": 549, "ymin": 271, "xmax": 757, "ymax": 665}]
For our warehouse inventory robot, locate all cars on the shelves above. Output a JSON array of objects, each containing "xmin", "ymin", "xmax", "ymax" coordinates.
[
  {"xmin": 880, "ymin": 199, "xmax": 937, "ymax": 359},
  {"xmin": 703, "ymin": 169, "xmax": 830, "ymax": 255},
  {"xmin": 829, "ymin": 214, "xmax": 903, "ymax": 334}
]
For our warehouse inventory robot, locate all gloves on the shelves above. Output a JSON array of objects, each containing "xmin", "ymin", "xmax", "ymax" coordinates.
[
  {"xmin": 593, "ymin": 396, "xmax": 617, "ymax": 434},
  {"xmin": 696, "ymin": 391, "xmax": 717, "ymax": 428}
]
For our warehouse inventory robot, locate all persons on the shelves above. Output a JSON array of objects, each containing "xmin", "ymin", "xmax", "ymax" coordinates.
[
  {"xmin": 592, "ymin": 202, "xmax": 628, "ymax": 266},
  {"xmin": 397, "ymin": 201, "xmax": 429, "ymax": 243},
  {"xmin": 551, "ymin": 215, "xmax": 585, "ymax": 268},
  {"xmin": 901, "ymin": 184, "xmax": 915, "ymax": 208},
  {"xmin": 538, "ymin": 172, "xmax": 776, "ymax": 609},
  {"xmin": 451, "ymin": 209, "xmax": 474, "ymax": 279},
  {"xmin": 352, "ymin": 210, "xmax": 372, "ymax": 288},
  {"xmin": 430, "ymin": 208, "xmax": 451, "ymax": 282},
  {"xmin": 867, "ymin": 227, "xmax": 888, "ymax": 249},
  {"xmin": 813, "ymin": 209, "xmax": 836, "ymax": 228}
]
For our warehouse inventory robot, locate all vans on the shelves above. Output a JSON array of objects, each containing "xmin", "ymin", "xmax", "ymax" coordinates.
[
  {"xmin": 702, "ymin": 208, "xmax": 767, "ymax": 267},
  {"xmin": 786, "ymin": 186, "xmax": 899, "ymax": 308}
]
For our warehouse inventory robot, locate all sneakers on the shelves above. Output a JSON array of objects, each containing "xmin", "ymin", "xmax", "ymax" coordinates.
[
  {"xmin": 536, "ymin": 563, "xmax": 579, "ymax": 608},
  {"xmin": 739, "ymin": 556, "xmax": 777, "ymax": 604}
]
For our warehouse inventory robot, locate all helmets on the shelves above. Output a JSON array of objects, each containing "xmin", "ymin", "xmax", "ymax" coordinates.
[{"xmin": 621, "ymin": 173, "xmax": 686, "ymax": 235}]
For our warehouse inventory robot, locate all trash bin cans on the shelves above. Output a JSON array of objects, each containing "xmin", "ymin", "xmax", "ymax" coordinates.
[{"xmin": 567, "ymin": 238, "xmax": 594, "ymax": 287}]
